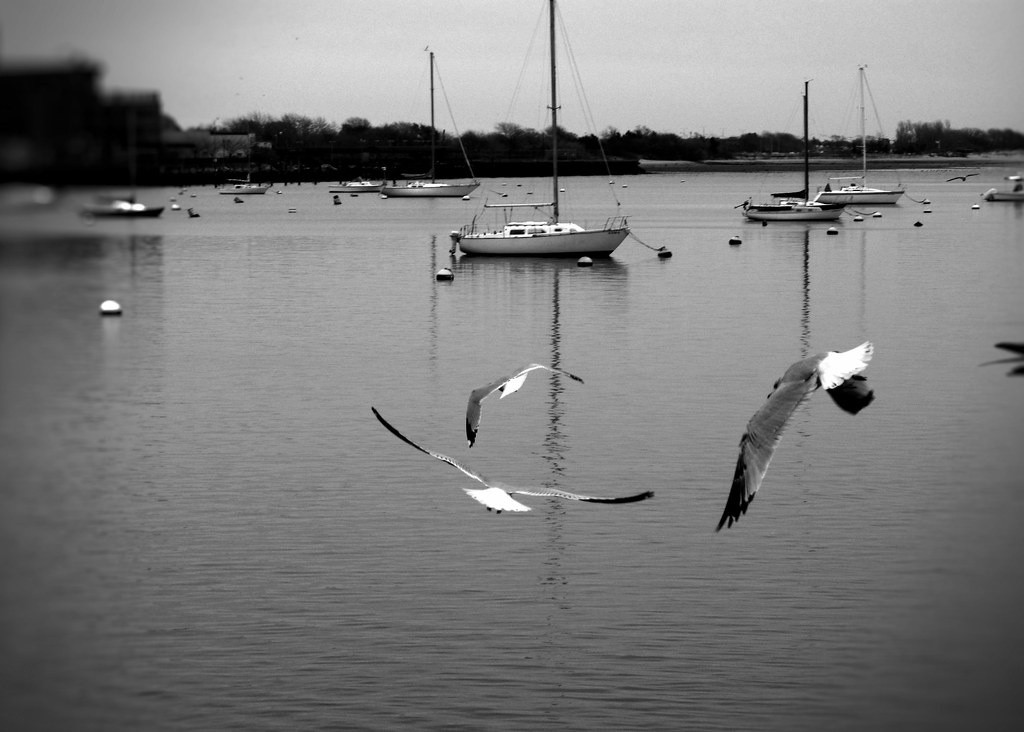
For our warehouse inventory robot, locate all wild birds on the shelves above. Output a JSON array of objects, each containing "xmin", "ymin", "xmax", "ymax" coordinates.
[
  {"xmin": 371, "ymin": 362, "xmax": 654, "ymax": 519},
  {"xmin": 717, "ymin": 341, "xmax": 875, "ymax": 531},
  {"xmin": 946, "ymin": 173, "xmax": 979, "ymax": 182}
]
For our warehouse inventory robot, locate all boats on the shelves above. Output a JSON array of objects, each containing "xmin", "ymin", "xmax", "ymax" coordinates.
[
  {"xmin": 84, "ymin": 198, "xmax": 165, "ymax": 218},
  {"xmin": 328, "ymin": 176, "xmax": 388, "ymax": 194},
  {"xmin": 983, "ymin": 174, "xmax": 1024, "ymax": 202},
  {"xmin": 219, "ymin": 172, "xmax": 273, "ymax": 195}
]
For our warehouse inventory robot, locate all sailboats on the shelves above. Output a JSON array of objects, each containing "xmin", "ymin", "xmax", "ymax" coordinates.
[
  {"xmin": 449, "ymin": 0, "xmax": 633, "ymax": 257},
  {"xmin": 733, "ymin": 75, "xmax": 853, "ymax": 222},
  {"xmin": 812, "ymin": 64, "xmax": 906, "ymax": 205},
  {"xmin": 379, "ymin": 51, "xmax": 481, "ymax": 198}
]
